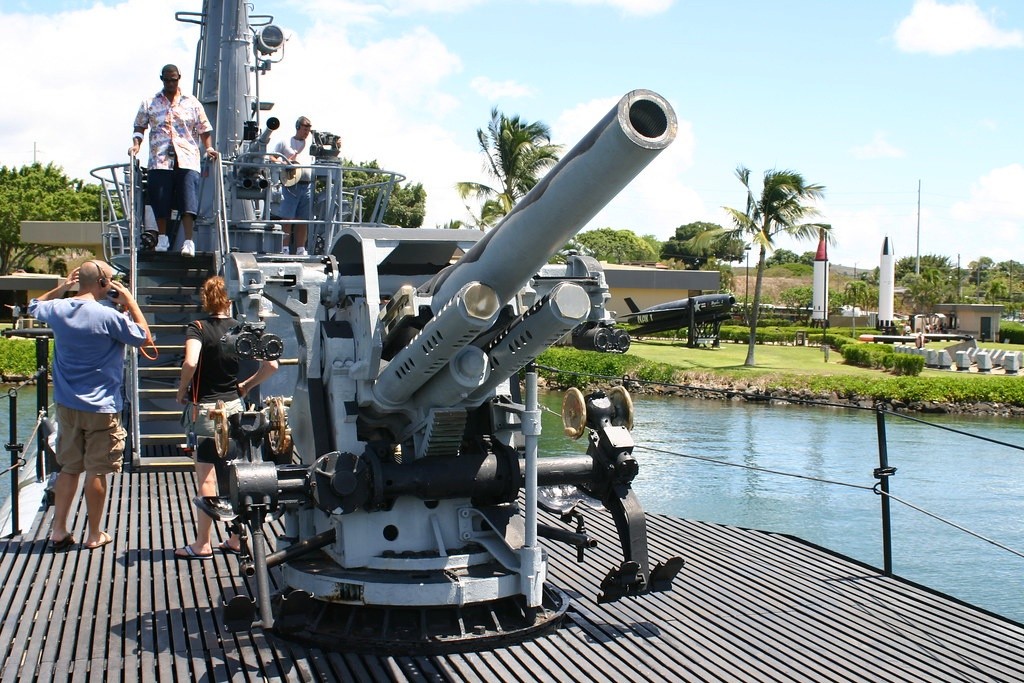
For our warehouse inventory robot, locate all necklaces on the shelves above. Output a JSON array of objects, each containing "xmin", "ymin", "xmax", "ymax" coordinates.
[{"xmin": 208, "ymin": 313, "xmax": 226, "ymax": 316}]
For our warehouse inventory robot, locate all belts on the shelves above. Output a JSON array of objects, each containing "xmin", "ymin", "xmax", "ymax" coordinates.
[{"xmin": 298, "ymin": 181, "xmax": 311, "ymax": 184}]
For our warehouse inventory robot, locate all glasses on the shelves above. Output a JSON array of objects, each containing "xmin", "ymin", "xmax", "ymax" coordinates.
[{"xmin": 162, "ymin": 77, "xmax": 179, "ymax": 81}]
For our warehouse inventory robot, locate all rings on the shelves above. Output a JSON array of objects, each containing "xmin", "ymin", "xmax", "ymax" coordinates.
[{"xmin": 176, "ymin": 399, "xmax": 179, "ymax": 401}]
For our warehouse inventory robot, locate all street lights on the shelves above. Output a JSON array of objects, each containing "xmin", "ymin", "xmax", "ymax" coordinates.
[{"xmin": 744, "ymin": 246, "xmax": 751, "ymax": 307}]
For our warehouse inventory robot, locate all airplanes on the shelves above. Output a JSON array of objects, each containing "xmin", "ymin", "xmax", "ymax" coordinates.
[{"xmin": 618, "ymin": 294, "xmax": 736, "ymax": 328}]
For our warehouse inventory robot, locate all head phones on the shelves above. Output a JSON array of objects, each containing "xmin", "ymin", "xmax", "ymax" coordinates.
[
  {"xmin": 295, "ymin": 117, "xmax": 304, "ymax": 130},
  {"xmin": 86, "ymin": 260, "xmax": 108, "ymax": 287}
]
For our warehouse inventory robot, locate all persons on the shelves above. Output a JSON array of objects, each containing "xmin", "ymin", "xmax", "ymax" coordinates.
[
  {"xmin": 903, "ymin": 320, "xmax": 949, "ymax": 344},
  {"xmin": 127, "ymin": 64, "xmax": 218, "ymax": 256},
  {"xmin": 269, "ymin": 116, "xmax": 341, "ymax": 255},
  {"xmin": 175, "ymin": 276, "xmax": 279, "ymax": 559},
  {"xmin": 27, "ymin": 259, "xmax": 151, "ymax": 551}
]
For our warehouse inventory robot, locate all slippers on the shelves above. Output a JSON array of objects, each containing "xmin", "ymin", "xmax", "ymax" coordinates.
[
  {"xmin": 48, "ymin": 533, "xmax": 74, "ymax": 548},
  {"xmin": 86, "ymin": 531, "xmax": 113, "ymax": 549},
  {"xmin": 176, "ymin": 545, "xmax": 213, "ymax": 559},
  {"xmin": 218, "ymin": 540, "xmax": 242, "ymax": 554}
]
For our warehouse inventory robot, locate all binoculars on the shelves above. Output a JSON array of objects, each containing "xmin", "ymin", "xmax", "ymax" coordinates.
[
  {"xmin": 575, "ymin": 323, "xmax": 632, "ymax": 354},
  {"xmin": 223, "ymin": 329, "xmax": 285, "ymax": 367}
]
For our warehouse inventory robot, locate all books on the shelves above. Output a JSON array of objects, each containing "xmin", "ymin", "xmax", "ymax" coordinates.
[{"xmin": 5, "ymin": 302, "xmax": 21, "ymax": 330}]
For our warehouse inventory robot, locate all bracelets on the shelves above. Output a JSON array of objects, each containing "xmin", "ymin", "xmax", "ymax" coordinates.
[
  {"xmin": 242, "ymin": 381, "xmax": 250, "ymax": 393},
  {"xmin": 205, "ymin": 146, "xmax": 214, "ymax": 151}
]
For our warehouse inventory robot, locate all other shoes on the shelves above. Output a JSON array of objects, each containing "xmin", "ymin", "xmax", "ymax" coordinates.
[
  {"xmin": 296, "ymin": 249, "xmax": 307, "ymax": 255},
  {"xmin": 282, "ymin": 247, "xmax": 289, "ymax": 254}
]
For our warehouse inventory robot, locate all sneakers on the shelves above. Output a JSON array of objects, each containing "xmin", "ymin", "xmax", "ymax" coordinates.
[
  {"xmin": 181, "ymin": 240, "xmax": 195, "ymax": 257},
  {"xmin": 155, "ymin": 236, "xmax": 170, "ymax": 252}
]
